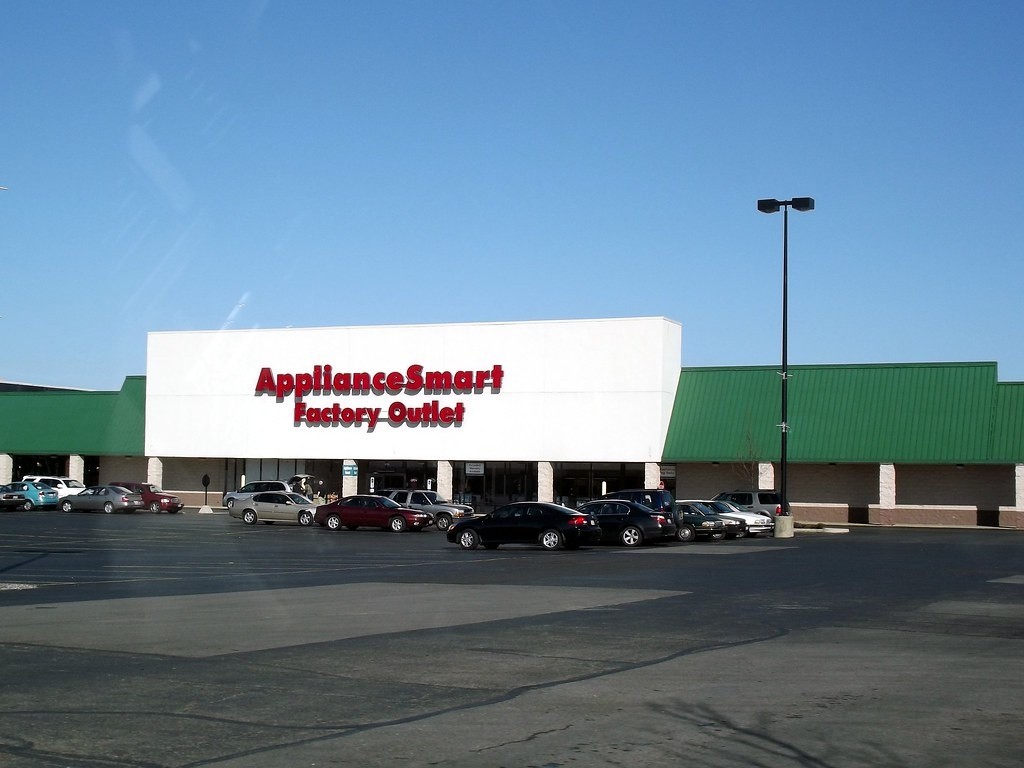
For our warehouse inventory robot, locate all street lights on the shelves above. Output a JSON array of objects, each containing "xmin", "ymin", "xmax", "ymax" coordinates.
[{"xmin": 758, "ymin": 198, "xmax": 814, "ymax": 518}]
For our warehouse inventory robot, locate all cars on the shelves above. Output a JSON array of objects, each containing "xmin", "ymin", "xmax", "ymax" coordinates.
[
  {"xmin": 448, "ymin": 501, "xmax": 602, "ymax": 550},
  {"xmin": 57, "ymin": 485, "xmax": 145, "ymax": 514},
  {"xmin": 5, "ymin": 481, "xmax": 58, "ymax": 510},
  {"xmin": 0, "ymin": 485, "xmax": 25, "ymax": 512},
  {"xmin": 574, "ymin": 499, "xmax": 675, "ymax": 547},
  {"xmin": 229, "ymin": 491, "xmax": 317, "ymax": 527},
  {"xmin": 314, "ymin": 495, "xmax": 429, "ymax": 531},
  {"xmin": 676, "ymin": 499, "xmax": 775, "ymax": 543}
]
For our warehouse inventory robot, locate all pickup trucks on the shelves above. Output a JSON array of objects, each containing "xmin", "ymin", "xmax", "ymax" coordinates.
[
  {"xmin": 375, "ymin": 489, "xmax": 473, "ymax": 531},
  {"xmin": 22, "ymin": 474, "xmax": 88, "ymax": 496}
]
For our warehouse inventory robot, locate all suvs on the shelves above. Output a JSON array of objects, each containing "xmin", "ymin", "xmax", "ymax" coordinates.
[
  {"xmin": 712, "ymin": 491, "xmax": 792, "ymax": 517},
  {"xmin": 600, "ymin": 490, "xmax": 679, "ymax": 527},
  {"xmin": 223, "ymin": 474, "xmax": 314, "ymax": 506},
  {"xmin": 111, "ymin": 481, "xmax": 183, "ymax": 514}
]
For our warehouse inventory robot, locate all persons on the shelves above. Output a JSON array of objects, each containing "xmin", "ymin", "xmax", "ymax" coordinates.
[
  {"xmin": 303, "ymin": 482, "xmax": 314, "ymax": 500},
  {"xmin": 293, "ymin": 479, "xmax": 304, "ymax": 494},
  {"xmin": 318, "ymin": 480, "xmax": 326, "ymax": 505},
  {"xmin": 329, "ymin": 491, "xmax": 339, "ymax": 504}
]
